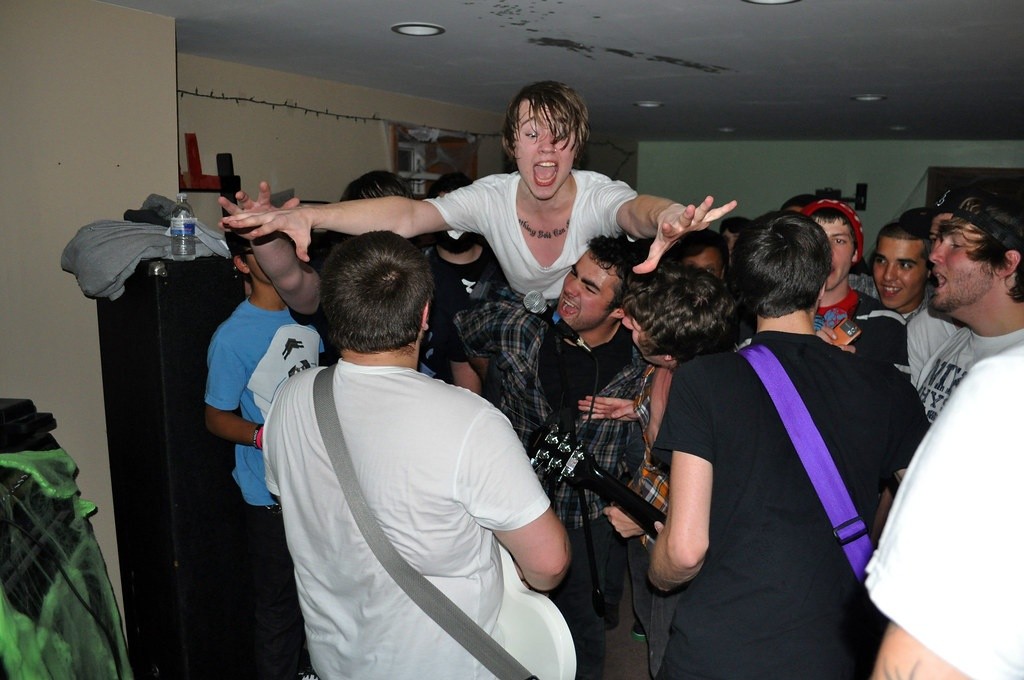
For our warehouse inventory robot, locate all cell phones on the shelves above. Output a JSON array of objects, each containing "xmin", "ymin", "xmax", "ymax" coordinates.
[{"xmin": 829, "ymin": 317, "xmax": 862, "ymax": 347}]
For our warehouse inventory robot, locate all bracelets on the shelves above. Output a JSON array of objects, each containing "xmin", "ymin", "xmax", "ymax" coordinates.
[
  {"xmin": 256, "ymin": 427, "xmax": 263, "ymax": 449},
  {"xmin": 253, "ymin": 424, "xmax": 263, "ymax": 449}
]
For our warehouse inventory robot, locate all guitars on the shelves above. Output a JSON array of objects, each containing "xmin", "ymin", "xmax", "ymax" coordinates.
[{"xmin": 526, "ymin": 420, "xmax": 668, "ymax": 540}]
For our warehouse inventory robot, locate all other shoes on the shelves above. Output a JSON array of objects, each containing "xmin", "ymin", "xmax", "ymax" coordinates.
[
  {"xmin": 631, "ymin": 619, "xmax": 647, "ymax": 641},
  {"xmin": 601, "ymin": 600, "xmax": 620, "ymax": 631}
]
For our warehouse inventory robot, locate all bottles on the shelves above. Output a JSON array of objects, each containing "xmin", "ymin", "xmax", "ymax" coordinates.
[{"xmin": 171, "ymin": 193, "xmax": 196, "ymax": 261}]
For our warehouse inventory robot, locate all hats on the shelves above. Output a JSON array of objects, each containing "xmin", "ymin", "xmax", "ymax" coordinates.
[
  {"xmin": 800, "ymin": 198, "xmax": 865, "ymax": 268},
  {"xmin": 898, "ymin": 180, "xmax": 1024, "ymax": 260}
]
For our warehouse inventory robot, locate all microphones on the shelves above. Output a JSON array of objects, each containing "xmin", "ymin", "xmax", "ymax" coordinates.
[{"xmin": 523, "ymin": 290, "xmax": 592, "ymax": 353}]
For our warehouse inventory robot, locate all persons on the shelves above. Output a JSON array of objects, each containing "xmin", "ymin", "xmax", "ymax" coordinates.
[
  {"xmin": 221, "ymin": 79, "xmax": 737, "ymax": 307},
  {"xmin": 202, "ymin": 168, "xmax": 1024, "ymax": 680}
]
